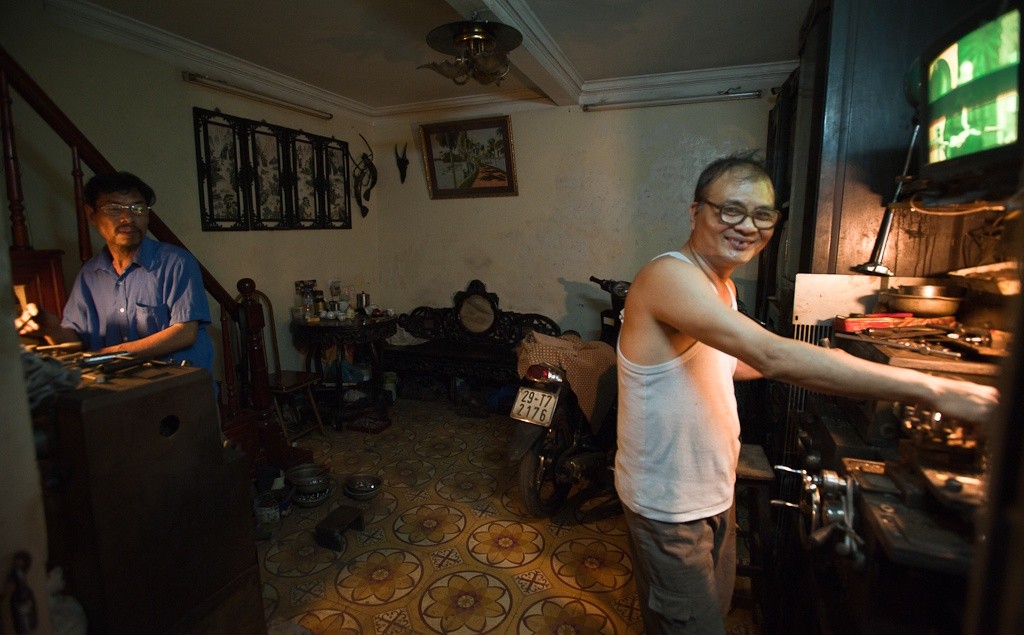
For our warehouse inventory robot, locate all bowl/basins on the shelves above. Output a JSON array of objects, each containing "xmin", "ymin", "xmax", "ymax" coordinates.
[
  {"xmin": 881, "ymin": 291, "xmax": 963, "ymax": 316},
  {"xmin": 343, "ymin": 474, "xmax": 382, "ymax": 499},
  {"xmin": 284, "ymin": 462, "xmax": 331, "ymax": 508},
  {"xmin": 898, "ymin": 285, "xmax": 968, "ymax": 298}
]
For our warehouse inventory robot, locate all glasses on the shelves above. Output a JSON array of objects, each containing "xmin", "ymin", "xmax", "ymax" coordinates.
[
  {"xmin": 701, "ymin": 199, "xmax": 780, "ymax": 229},
  {"xmin": 97, "ymin": 201, "xmax": 152, "ymax": 216}
]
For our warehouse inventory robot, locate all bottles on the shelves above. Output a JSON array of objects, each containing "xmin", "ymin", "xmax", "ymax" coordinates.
[
  {"xmin": 304, "ymin": 294, "xmax": 313, "ymax": 321},
  {"xmin": 356, "ymin": 291, "xmax": 369, "ymax": 312}
]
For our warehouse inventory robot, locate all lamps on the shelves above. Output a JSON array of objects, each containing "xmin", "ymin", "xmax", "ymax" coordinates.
[
  {"xmin": 416, "ymin": 9, "xmax": 523, "ymax": 87},
  {"xmin": 583, "ymin": 90, "xmax": 760, "ymax": 112},
  {"xmin": 183, "ymin": 71, "xmax": 332, "ymax": 120}
]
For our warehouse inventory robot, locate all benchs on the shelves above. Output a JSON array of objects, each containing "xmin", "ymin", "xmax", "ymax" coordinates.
[{"xmin": 384, "ymin": 280, "xmax": 581, "ymax": 416}]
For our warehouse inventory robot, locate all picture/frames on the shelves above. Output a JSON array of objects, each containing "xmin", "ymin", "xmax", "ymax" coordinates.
[{"xmin": 420, "ymin": 114, "xmax": 518, "ymax": 200}]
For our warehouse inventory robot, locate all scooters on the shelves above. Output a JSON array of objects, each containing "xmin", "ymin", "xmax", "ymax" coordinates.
[{"xmin": 506, "ymin": 275, "xmax": 631, "ymax": 518}]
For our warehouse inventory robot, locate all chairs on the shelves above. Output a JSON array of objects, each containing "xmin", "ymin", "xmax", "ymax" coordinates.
[{"xmin": 226, "ymin": 291, "xmax": 328, "ymax": 446}]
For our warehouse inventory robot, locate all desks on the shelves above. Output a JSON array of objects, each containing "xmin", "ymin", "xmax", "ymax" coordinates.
[
  {"xmin": 18, "ymin": 342, "xmax": 270, "ymax": 635},
  {"xmin": 290, "ymin": 311, "xmax": 398, "ymax": 425}
]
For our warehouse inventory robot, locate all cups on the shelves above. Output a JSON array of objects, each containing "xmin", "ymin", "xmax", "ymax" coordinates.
[
  {"xmin": 336, "ymin": 312, "xmax": 346, "ymax": 320},
  {"xmin": 387, "ymin": 309, "xmax": 395, "ymax": 317},
  {"xmin": 327, "ymin": 311, "xmax": 336, "ymax": 319},
  {"xmin": 339, "ymin": 302, "xmax": 349, "ymax": 312}
]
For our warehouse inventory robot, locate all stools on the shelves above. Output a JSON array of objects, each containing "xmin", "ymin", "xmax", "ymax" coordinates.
[
  {"xmin": 737, "ymin": 444, "xmax": 778, "ymax": 625},
  {"xmin": 316, "ymin": 505, "xmax": 365, "ymax": 549}
]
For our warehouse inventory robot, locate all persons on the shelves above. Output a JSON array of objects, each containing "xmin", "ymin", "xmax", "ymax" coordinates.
[
  {"xmin": 16, "ymin": 172, "xmax": 218, "ymax": 423},
  {"xmin": 614, "ymin": 157, "xmax": 1001, "ymax": 635}
]
